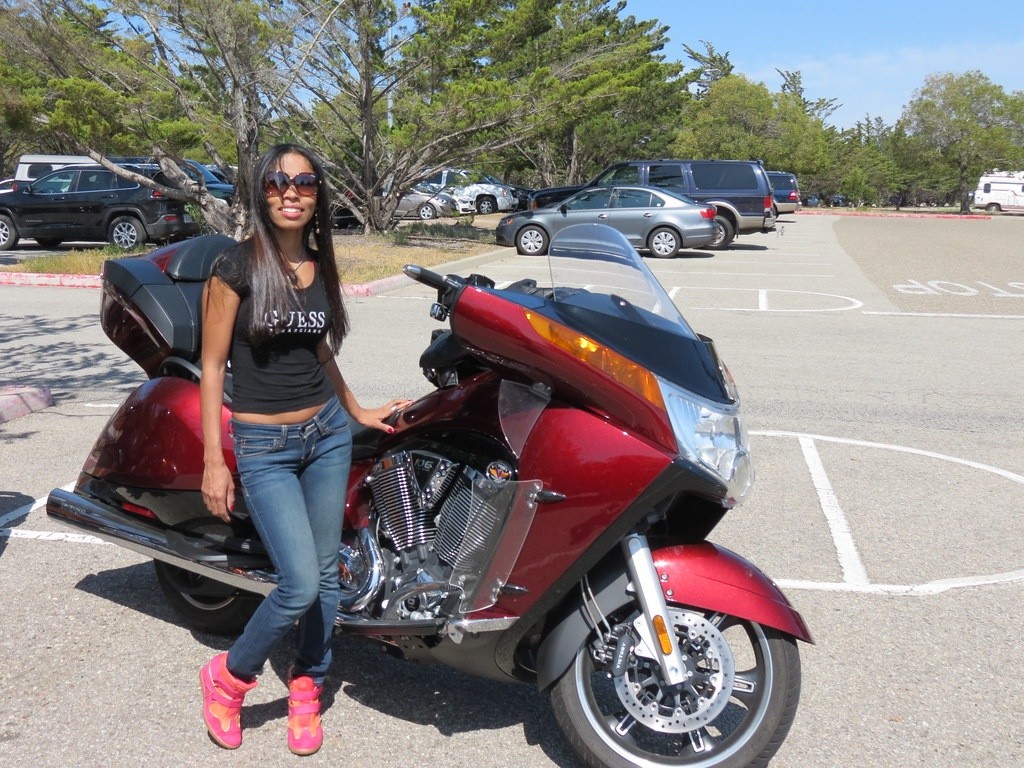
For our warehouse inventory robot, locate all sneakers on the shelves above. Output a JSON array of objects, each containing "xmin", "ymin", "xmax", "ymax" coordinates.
[
  {"xmin": 287, "ymin": 676, "xmax": 323, "ymax": 758},
  {"xmin": 199, "ymin": 652, "xmax": 259, "ymax": 751}
]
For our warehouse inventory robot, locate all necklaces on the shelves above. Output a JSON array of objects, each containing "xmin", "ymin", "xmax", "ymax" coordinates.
[{"xmin": 290, "ymin": 245, "xmax": 306, "ymax": 272}]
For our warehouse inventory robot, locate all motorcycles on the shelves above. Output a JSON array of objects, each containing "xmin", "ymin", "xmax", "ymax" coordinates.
[{"xmin": 45, "ymin": 221, "xmax": 818, "ymax": 768}]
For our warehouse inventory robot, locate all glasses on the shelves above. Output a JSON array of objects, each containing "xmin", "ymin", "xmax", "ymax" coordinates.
[{"xmin": 260, "ymin": 170, "xmax": 324, "ymax": 196}]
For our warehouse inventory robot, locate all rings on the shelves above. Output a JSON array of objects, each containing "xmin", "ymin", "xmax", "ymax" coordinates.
[{"xmin": 390, "ymin": 404, "xmax": 398, "ymax": 413}]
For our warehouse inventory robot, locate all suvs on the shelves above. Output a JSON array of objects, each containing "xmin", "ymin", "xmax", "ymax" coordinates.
[
  {"xmin": 0, "ymin": 162, "xmax": 202, "ymax": 252},
  {"xmin": 526, "ymin": 159, "xmax": 778, "ymax": 251},
  {"xmin": 0, "ymin": 154, "xmax": 238, "ymax": 206}
]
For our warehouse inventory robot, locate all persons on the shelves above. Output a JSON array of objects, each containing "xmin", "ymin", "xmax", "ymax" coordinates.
[{"xmin": 202, "ymin": 143, "xmax": 415, "ymax": 757}]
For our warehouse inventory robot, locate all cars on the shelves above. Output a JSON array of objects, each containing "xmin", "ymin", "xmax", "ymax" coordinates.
[
  {"xmin": 508, "ymin": 182, "xmax": 535, "ymax": 212},
  {"xmin": 429, "ymin": 182, "xmax": 479, "ymax": 217},
  {"xmin": 495, "ymin": 182, "xmax": 720, "ymax": 259},
  {"xmin": 380, "ymin": 179, "xmax": 461, "ymax": 222},
  {"xmin": 829, "ymin": 193, "xmax": 847, "ymax": 207},
  {"xmin": 804, "ymin": 194, "xmax": 820, "ymax": 207}
]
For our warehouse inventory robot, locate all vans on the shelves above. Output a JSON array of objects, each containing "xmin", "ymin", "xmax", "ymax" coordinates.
[
  {"xmin": 425, "ymin": 168, "xmax": 520, "ymax": 216},
  {"xmin": 973, "ymin": 168, "xmax": 1024, "ymax": 216},
  {"xmin": 763, "ymin": 169, "xmax": 804, "ymax": 214}
]
